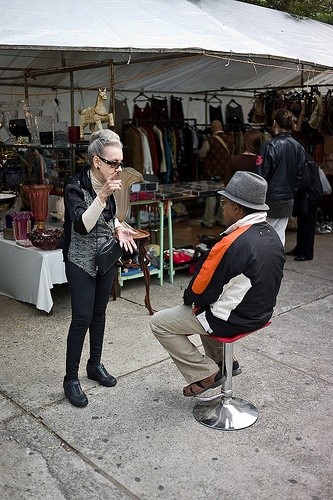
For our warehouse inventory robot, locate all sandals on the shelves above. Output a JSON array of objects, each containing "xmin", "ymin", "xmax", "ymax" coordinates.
[
  {"xmin": 216, "ymin": 358, "xmax": 242, "ymax": 377},
  {"xmin": 183, "ymin": 370, "xmax": 226, "ymax": 396}
]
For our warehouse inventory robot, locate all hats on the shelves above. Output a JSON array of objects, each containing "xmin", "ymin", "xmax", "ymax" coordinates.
[{"xmin": 218, "ymin": 171, "xmax": 270, "ymax": 210}]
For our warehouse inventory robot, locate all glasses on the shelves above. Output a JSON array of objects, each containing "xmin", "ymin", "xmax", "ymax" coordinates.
[
  {"xmin": 98, "ymin": 156, "xmax": 124, "ymax": 169},
  {"xmin": 219, "ymin": 200, "xmax": 231, "ymax": 207}
]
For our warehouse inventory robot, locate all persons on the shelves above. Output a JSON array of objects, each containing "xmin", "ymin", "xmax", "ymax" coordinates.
[
  {"xmin": 149, "ymin": 171, "xmax": 285, "ymax": 397},
  {"xmin": 199, "ymin": 121, "xmax": 233, "ymax": 229},
  {"xmin": 286, "ymin": 161, "xmax": 323, "ymax": 261},
  {"xmin": 63, "ymin": 129, "xmax": 139, "ymax": 408},
  {"xmin": 232, "ymin": 110, "xmax": 306, "ymax": 254}
]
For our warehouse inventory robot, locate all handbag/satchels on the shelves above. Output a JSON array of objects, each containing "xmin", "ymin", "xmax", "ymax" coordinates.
[
  {"xmin": 95, "ymin": 239, "xmax": 123, "ymax": 275},
  {"xmin": 318, "ymin": 168, "xmax": 331, "ymax": 194}
]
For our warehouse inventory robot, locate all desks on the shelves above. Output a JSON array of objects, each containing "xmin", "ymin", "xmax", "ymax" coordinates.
[{"xmin": 0, "ymin": 224, "xmax": 68, "ymax": 316}]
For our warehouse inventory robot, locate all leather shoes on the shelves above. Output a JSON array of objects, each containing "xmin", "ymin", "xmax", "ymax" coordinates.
[
  {"xmin": 63, "ymin": 375, "xmax": 88, "ymax": 407},
  {"xmin": 86, "ymin": 359, "xmax": 117, "ymax": 387}
]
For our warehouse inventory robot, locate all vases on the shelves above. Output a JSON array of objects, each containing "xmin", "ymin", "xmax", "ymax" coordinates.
[
  {"xmin": 0, "ymin": 100, "xmax": 46, "ymax": 145},
  {"xmin": 23, "ymin": 184, "xmax": 54, "ymax": 230}
]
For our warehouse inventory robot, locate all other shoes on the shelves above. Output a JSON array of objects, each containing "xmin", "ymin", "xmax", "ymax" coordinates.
[
  {"xmin": 294, "ymin": 256, "xmax": 309, "ymax": 261},
  {"xmin": 285, "ymin": 248, "xmax": 298, "ymax": 256},
  {"xmin": 200, "ymin": 222, "xmax": 212, "ymax": 229}
]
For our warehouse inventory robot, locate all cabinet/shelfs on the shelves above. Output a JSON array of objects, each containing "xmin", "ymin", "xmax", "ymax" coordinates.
[
  {"xmin": 55, "ymin": 148, "xmax": 72, "ymax": 181},
  {"xmin": 118, "ymin": 192, "xmax": 218, "ymax": 287}
]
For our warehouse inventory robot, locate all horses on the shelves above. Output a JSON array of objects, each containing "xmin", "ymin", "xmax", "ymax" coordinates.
[{"xmin": 78, "ymin": 88, "xmax": 114, "ymax": 141}]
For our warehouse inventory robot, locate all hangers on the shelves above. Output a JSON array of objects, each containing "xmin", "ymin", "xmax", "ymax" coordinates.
[
  {"xmin": 115, "ymin": 89, "xmax": 241, "ymax": 107},
  {"xmin": 248, "ymin": 84, "xmax": 333, "ymax": 104}
]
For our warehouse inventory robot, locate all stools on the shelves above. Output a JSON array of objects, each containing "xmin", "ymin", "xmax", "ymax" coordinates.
[{"xmin": 192, "ymin": 322, "xmax": 272, "ymax": 430}]
